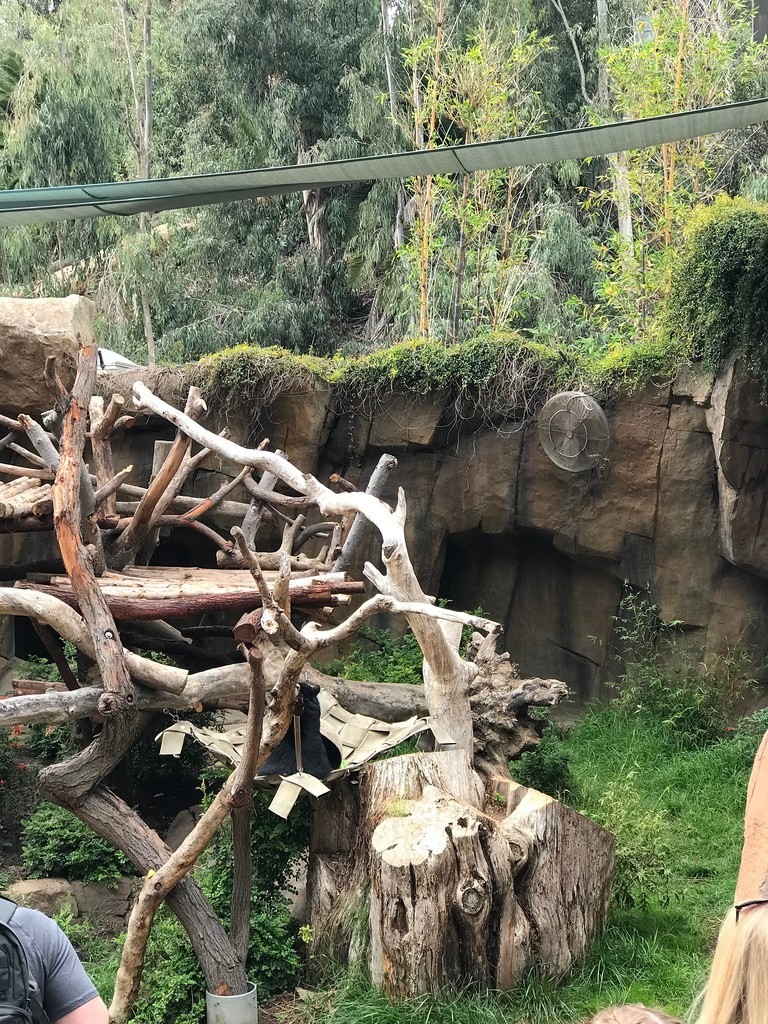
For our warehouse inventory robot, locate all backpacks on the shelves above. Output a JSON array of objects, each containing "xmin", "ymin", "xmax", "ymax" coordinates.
[{"xmin": 0, "ymin": 898, "xmax": 53, "ymax": 1024}]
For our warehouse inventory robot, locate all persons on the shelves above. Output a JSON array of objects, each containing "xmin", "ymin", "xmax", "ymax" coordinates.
[
  {"xmin": 0, "ymin": 895, "xmax": 109, "ymax": 1024},
  {"xmin": 691, "ymin": 898, "xmax": 768, "ymax": 1024}
]
